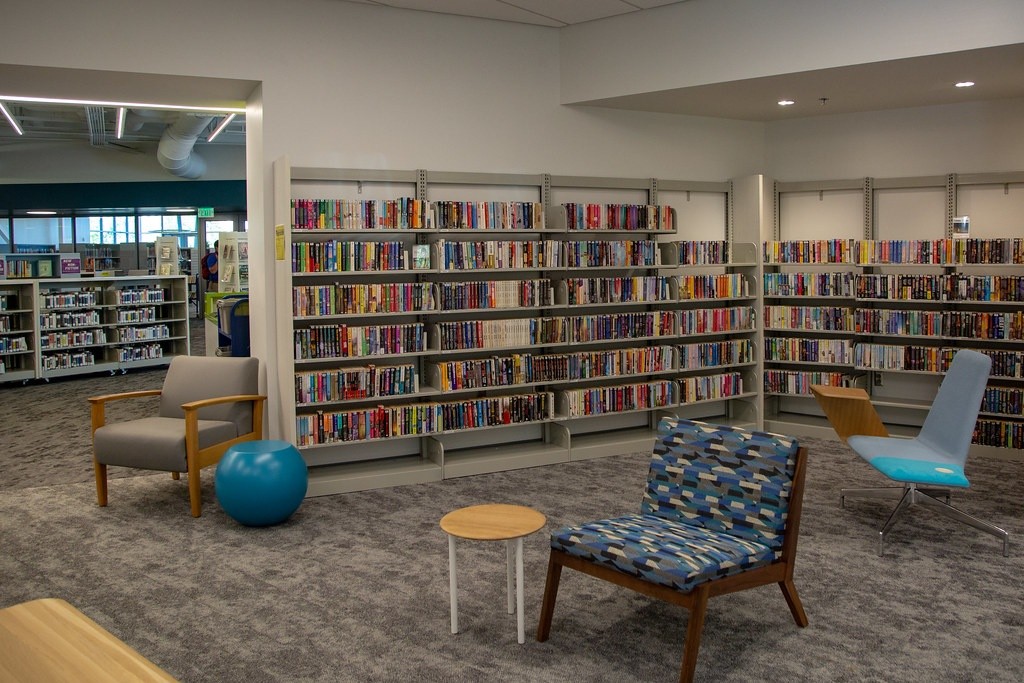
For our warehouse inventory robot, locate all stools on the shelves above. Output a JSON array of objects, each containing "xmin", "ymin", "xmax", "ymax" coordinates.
[{"xmin": 215, "ymin": 440, "xmax": 309, "ymax": 532}]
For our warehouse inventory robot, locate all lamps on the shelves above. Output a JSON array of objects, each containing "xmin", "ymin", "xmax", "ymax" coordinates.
[{"xmin": 0, "ymin": 95, "xmax": 246, "ymax": 143}]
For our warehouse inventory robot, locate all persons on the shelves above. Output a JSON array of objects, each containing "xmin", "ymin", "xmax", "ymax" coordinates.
[{"xmin": 207, "ymin": 240, "xmax": 218, "ymax": 292}]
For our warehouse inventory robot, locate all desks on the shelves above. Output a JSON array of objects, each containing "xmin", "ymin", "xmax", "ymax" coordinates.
[{"xmin": 441, "ymin": 501, "xmax": 548, "ymax": 644}]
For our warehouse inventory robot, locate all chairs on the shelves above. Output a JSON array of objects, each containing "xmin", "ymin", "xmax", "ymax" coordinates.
[
  {"xmin": 535, "ymin": 416, "xmax": 809, "ymax": 683},
  {"xmin": 88, "ymin": 354, "xmax": 268, "ymax": 518},
  {"xmin": 810, "ymin": 345, "xmax": 1011, "ymax": 557}
]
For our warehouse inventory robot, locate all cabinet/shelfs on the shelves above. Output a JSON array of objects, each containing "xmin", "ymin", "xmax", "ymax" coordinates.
[
  {"xmin": 762, "ymin": 170, "xmax": 1024, "ymax": 463},
  {"xmin": 0, "ymin": 232, "xmax": 250, "ymax": 391},
  {"xmin": 274, "ymin": 151, "xmax": 762, "ymax": 496}
]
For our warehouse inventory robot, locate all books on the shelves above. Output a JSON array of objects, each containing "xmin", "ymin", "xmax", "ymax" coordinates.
[
  {"xmin": 146, "ymin": 247, "xmax": 156, "ymax": 274},
  {"xmin": 291, "ymin": 198, "xmax": 752, "ymax": 446},
  {"xmin": 763, "ymin": 238, "xmax": 1024, "ymax": 450},
  {"xmin": 7, "ymin": 260, "xmax": 27, "ymax": 277},
  {"xmin": 0, "ymin": 288, "xmax": 169, "ymax": 370},
  {"xmin": 85, "ymin": 248, "xmax": 113, "ymax": 271}
]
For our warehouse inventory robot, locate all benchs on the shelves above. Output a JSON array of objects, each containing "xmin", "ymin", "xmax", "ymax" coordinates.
[{"xmin": 0, "ymin": 598, "xmax": 184, "ymax": 683}]
